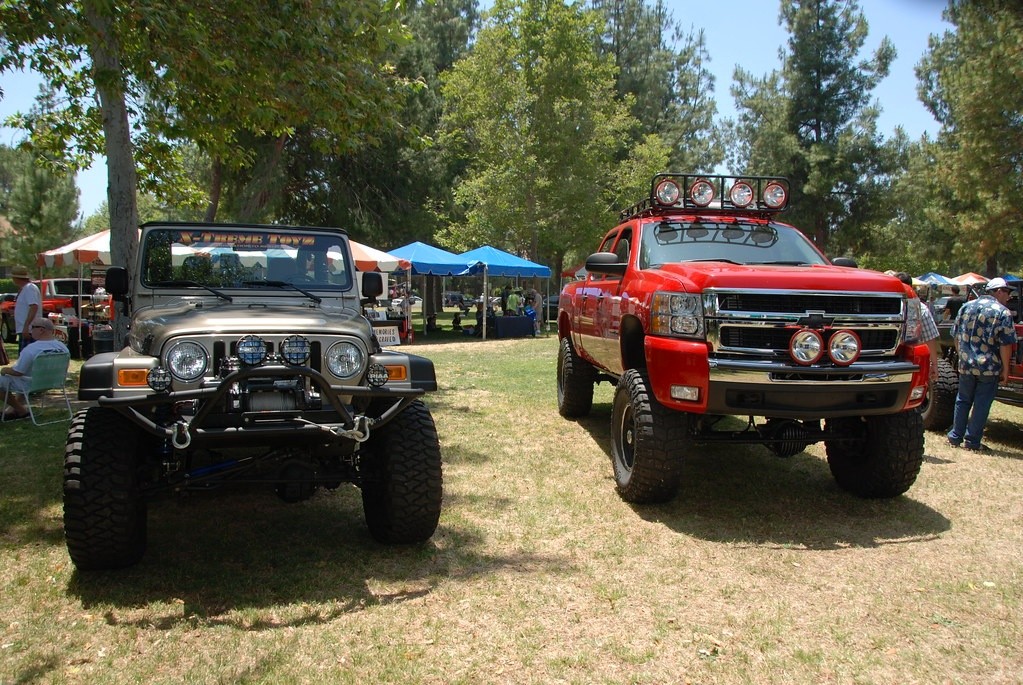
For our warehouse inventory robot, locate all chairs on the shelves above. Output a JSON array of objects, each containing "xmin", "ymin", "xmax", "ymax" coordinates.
[
  {"xmin": 267, "ymin": 255, "xmax": 307, "ymax": 284},
  {"xmin": 0, "ymin": 351, "xmax": 74, "ymax": 427},
  {"xmin": 181, "ymin": 256, "xmax": 222, "ymax": 285}
]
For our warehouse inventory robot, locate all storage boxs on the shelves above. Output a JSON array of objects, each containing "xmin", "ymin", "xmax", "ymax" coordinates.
[{"xmin": 463, "ymin": 325, "xmax": 475, "ymax": 335}]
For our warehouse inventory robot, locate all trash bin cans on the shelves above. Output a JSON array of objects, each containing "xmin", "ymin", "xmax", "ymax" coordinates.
[
  {"xmin": 92, "ymin": 330, "xmax": 115, "ymax": 353},
  {"xmin": 67, "ymin": 326, "xmax": 89, "ymax": 356}
]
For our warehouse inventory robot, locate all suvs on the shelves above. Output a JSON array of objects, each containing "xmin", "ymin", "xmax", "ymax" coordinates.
[
  {"xmin": 920, "ymin": 316, "xmax": 1022, "ymax": 434},
  {"xmin": 61, "ymin": 219, "xmax": 444, "ymax": 570},
  {"xmin": 553, "ymin": 172, "xmax": 937, "ymax": 508},
  {"xmin": 33, "ymin": 277, "xmax": 114, "ymax": 321},
  {"xmin": 442, "ymin": 293, "xmax": 462, "ymax": 307}
]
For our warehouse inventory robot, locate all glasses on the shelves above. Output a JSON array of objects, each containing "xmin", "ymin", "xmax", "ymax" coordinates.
[
  {"xmin": 1002, "ymin": 289, "xmax": 1011, "ymax": 295},
  {"xmin": 32, "ymin": 325, "xmax": 46, "ymax": 329}
]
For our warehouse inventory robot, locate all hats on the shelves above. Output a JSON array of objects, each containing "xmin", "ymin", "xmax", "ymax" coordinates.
[
  {"xmin": 513, "ymin": 287, "xmax": 521, "ymax": 291},
  {"xmin": 9, "ymin": 266, "xmax": 29, "ymax": 278},
  {"xmin": 985, "ymin": 277, "xmax": 1017, "ymax": 292}
]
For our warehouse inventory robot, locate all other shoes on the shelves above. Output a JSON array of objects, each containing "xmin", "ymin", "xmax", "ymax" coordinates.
[
  {"xmin": 1, "ymin": 409, "xmax": 31, "ymax": 421},
  {"xmin": 947, "ymin": 432, "xmax": 961, "ymax": 447},
  {"xmin": 535, "ymin": 331, "xmax": 541, "ymax": 334},
  {"xmin": 964, "ymin": 444, "xmax": 993, "ymax": 451}
]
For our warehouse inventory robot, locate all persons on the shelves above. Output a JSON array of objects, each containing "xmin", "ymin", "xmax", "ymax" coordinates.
[
  {"xmin": 7, "ymin": 265, "xmax": 42, "ymax": 356},
  {"xmin": 944, "ymin": 285, "xmax": 967, "ymax": 323},
  {"xmin": 425, "ymin": 283, "xmax": 544, "ymax": 335},
  {"xmin": 0, "ymin": 316, "xmax": 70, "ymax": 422},
  {"xmin": 895, "ymin": 272, "xmax": 940, "ymax": 382},
  {"xmin": 947, "ymin": 278, "xmax": 1019, "ymax": 452}
]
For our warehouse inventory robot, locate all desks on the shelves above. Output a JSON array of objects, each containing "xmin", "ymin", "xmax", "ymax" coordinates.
[
  {"xmin": 53, "ymin": 325, "xmax": 89, "ymax": 358},
  {"xmin": 494, "ymin": 315, "xmax": 535, "ymax": 339}
]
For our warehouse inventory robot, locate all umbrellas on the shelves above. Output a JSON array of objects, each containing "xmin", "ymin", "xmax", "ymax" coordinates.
[{"xmin": 884, "ymin": 269, "xmax": 1023, "ymax": 308}]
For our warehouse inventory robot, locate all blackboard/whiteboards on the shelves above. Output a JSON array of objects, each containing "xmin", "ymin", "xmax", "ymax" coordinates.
[{"xmin": 91, "ymin": 270, "xmax": 106, "ymax": 293}]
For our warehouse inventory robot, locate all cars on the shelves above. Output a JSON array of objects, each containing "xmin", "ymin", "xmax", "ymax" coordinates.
[
  {"xmin": 388, "ymin": 278, "xmax": 420, "ymax": 290},
  {"xmin": 933, "ymin": 297, "xmax": 951, "ymax": 312},
  {"xmin": 0, "ymin": 293, "xmax": 20, "ymax": 341},
  {"xmin": 543, "ymin": 295, "xmax": 560, "ymax": 321},
  {"xmin": 464, "ymin": 296, "xmax": 503, "ymax": 307},
  {"xmin": 391, "ymin": 296, "xmax": 423, "ymax": 310}
]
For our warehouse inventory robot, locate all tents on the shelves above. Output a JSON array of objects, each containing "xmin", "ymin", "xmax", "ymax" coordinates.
[
  {"xmin": 386, "ymin": 241, "xmax": 486, "ymax": 343},
  {"xmin": 559, "ymin": 261, "xmax": 587, "ymax": 292},
  {"xmin": 189, "ymin": 233, "xmax": 304, "ymax": 284},
  {"xmin": 307, "ymin": 239, "xmax": 412, "ymax": 342},
  {"xmin": 457, "ymin": 246, "xmax": 552, "ymax": 338},
  {"xmin": 36, "ymin": 224, "xmax": 196, "ymax": 360}
]
